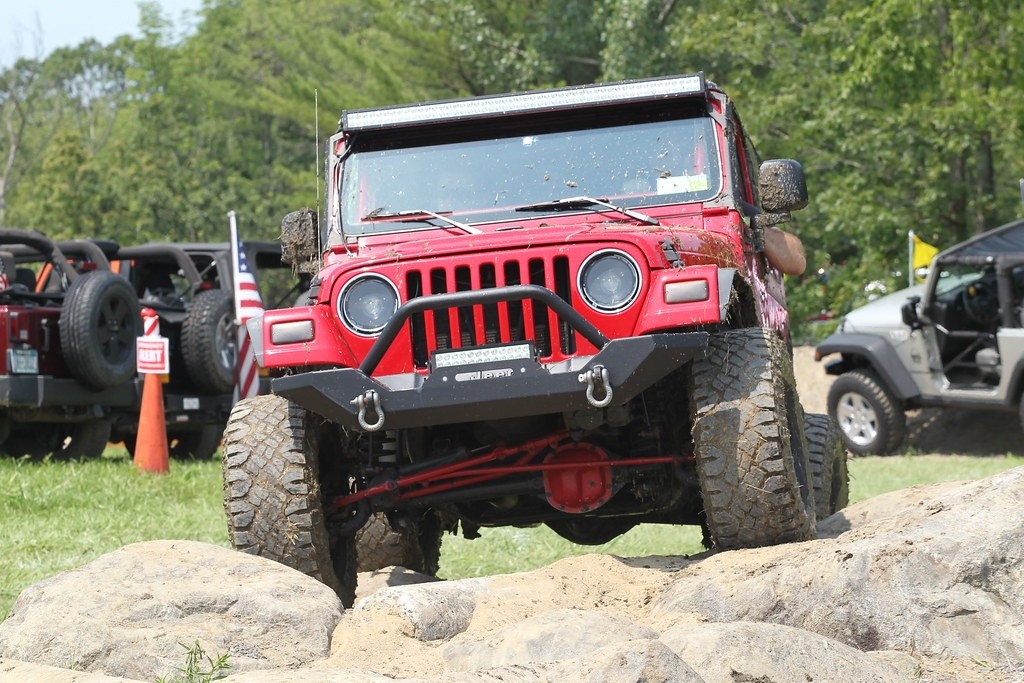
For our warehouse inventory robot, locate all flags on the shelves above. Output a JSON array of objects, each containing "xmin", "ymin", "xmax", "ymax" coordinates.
[{"xmin": 232, "ymin": 234, "xmax": 264, "ymax": 410}]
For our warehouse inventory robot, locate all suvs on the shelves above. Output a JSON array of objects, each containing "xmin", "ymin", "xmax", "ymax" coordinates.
[
  {"xmin": 220, "ymin": 71, "xmax": 849, "ymax": 608},
  {"xmin": 1, "ymin": 230, "xmax": 312, "ymax": 463},
  {"xmin": 814, "ymin": 216, "xmax": 1024, "ymax": 460}
]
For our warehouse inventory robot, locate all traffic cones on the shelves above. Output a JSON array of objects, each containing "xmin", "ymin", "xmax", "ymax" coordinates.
[{"xmin": 128, "ymin": 372, "xmax": 172, "ymax": 477}]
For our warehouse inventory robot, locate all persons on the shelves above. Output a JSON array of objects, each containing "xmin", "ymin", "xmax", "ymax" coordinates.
[{"xmin": 742, "ymin": 201, "xmax": 853, "ymax": 321}]
